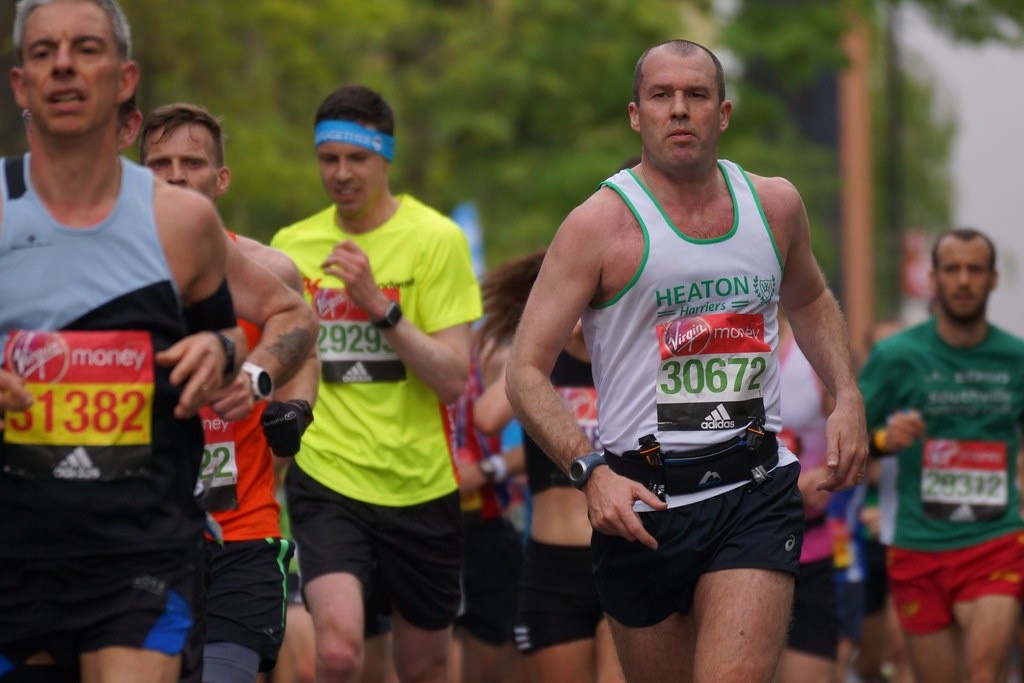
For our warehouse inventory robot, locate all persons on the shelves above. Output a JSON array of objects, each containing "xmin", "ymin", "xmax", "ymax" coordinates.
[
  {"xmin": 503, "ymin": 40, "xmax": 868, "ymax": 683},
  {"xmin": 855, "ymin": 229, "xmax": 1024, "ymax": 683},
  {"xmin": 0, "ymin": 0, "xmax": 246, "ymax": 683},
  {"xmin": 777, "ymin": 299, "xmax": 915, "ymax": 683},
  {"xmin": 270, "ymin": 85, "xmax": 483, "ymax": 683},
  {"xmin": 440, "ymin": 335, "xmax": 527, "ymax": 683},
  {"xmin": 138, "ymin": 104, "xmax": 321, "ymax": 683},
  {"xmin": 473, "ymin": 248, "xmax": 627, "ymax": 683}
]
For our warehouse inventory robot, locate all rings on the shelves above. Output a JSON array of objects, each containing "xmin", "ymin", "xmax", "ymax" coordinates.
[
  {"xmin": 858, "ymin": 473, "xmax": 864, "ymax": 478},
  {"xmin": 202, "ymin": 385, "xmax": 209, "ymax": 392}
]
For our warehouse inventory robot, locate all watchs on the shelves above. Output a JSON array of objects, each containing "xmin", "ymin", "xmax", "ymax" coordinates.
[
  {"xmin": 569, "ymin": 453, "xmax": 606, "ymax": 491},
  {"xmin": 373, "ymin": 301, "xmax": 402, "ymax": 329},
  {"xmin": 240, "ymin": 362, "xmax": 272, "ymax": 401},
  {"xmin": 289, "ymin": 399, "xmax": 314, "ymax": 427}
]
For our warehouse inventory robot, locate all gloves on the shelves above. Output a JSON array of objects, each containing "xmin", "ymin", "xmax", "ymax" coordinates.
[{"xmin": 260, "ymin": 399, "xmax": 314, "ymax": 457}]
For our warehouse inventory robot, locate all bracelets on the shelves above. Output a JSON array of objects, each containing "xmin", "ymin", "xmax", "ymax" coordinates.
[
  {"xmin": 216, "ymin": 332, "xmax": 235, "ymax": 389},
  {"xmin": 875, "ymin": 431, "xmax": 887, "ymax": 449}
]
